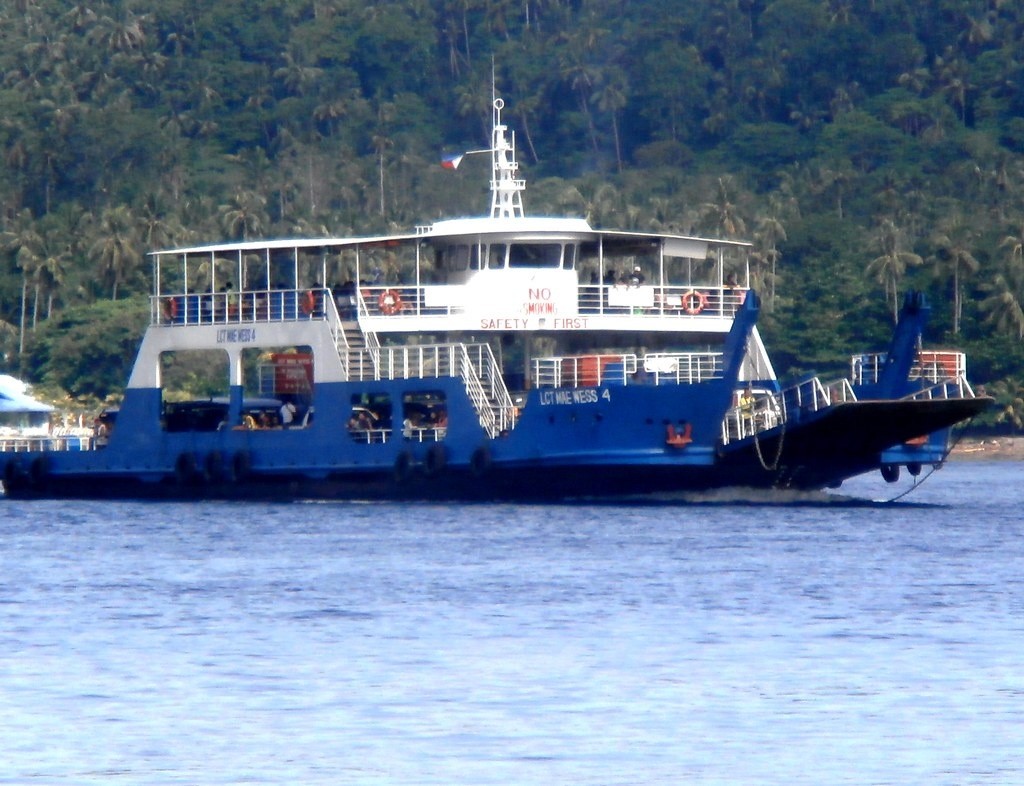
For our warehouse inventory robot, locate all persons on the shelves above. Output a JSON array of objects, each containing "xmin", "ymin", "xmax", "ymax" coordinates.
[
  {"xmin": 494, "ymin": 407, "xmax": 524, "ymax": 439},
  {"xmin": 348, "ymin": 411, "xmax": 387, "ymax": 444},
  {"xmin": 230, "ymin": 398, "xmax": 297, "ymax": 430},
  {"xmin": 739, "ymin": 389, "xmax": 758, "ymax": 439},
  {"xmin": 93, "ymin": 418, "xmax": 110, "ymax": 450},
  {"xmin": 582, "ymin": 266, "xmax": 646, "ymax": 306},
  {"xmin": 700, "ymin": 275, "xmax": 739, "ymax": 314},
  {"xmin": 187, "ymin": 272, "xmax": 406, "ymax": 323},
  {"xmin": 402, "ymin": 410, "xmax": 448, "ymax": 442}
]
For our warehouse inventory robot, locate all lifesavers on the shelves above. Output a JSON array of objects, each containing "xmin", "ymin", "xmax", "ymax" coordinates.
[
  {"xmin": 301, "ymin": 291, "xmax": 315, "ymax": 316},
  {"xmin": 203, "ymin": 451, "xmax": 223, "ymax": 483},
  {"xmin": 175, "ymin": 453, "xmax": 196, "ymax": 484},
  {"xmin": 378, "ymin": 290, "xmax": 402, "ymax": 315},
  {"xmin": 682, "ymin": 289, "xmax": 705, "ymax": 315},
  {"xmin": 906, "ymin": 463, "xmax": 923, "ymax": 475},
  {"xmin": 231, "ymin": 450, "xmax": 250, "ymax": 482},
  {"xmin": 3, "ymin": 459, "xmax": 21, "ymax": 487},
  {"xmin": 423, "ymin": 442, "xmax": 444, "ymax": 475},
  {"xmin": 469, "ymin": 448, "xmax": 492, "ymax": 480},
  {"xmin": 28, "ymin": 456, "xmax": 49, "ymax": 480},
  {"xmin": 880, "ymin": 462, "xmax": 900, "ymax": 482},
  {"xmin": 393, "ymin": 451, "xmax": 413, "ymax": 486},
  {"xmin": 163, "ymin": 297, "xmax": 177, "ymax": 320}
]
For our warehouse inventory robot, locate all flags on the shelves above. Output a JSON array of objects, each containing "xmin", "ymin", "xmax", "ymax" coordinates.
[{"xmin": 439, "ymin": 151, "xmax": 466, "ymax": 171}]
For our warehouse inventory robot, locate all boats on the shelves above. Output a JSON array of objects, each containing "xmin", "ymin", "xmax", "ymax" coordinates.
[{"xmin": 0, "ymin": 49, "xmax": 1000, "ymax": 505}]
